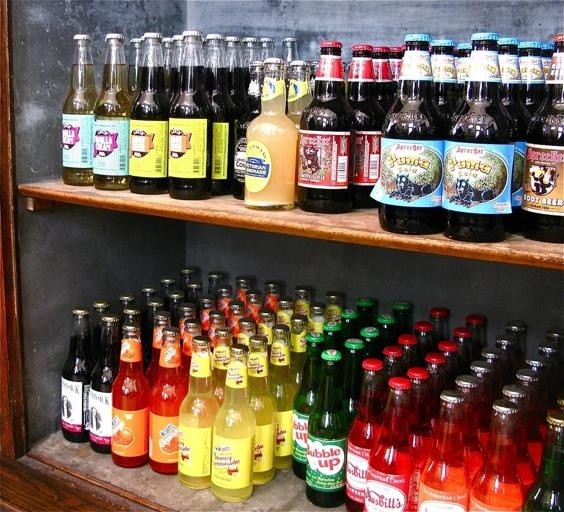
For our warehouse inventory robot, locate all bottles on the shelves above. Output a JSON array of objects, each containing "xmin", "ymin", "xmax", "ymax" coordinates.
[
  {"xmin": 494, "ymin": 38, "xmax": 531, "ymax": 235},
  {"xmin": 177, "ymin": 336, "xmax": 219, "ymax": 489},
  {"xmin": 322, "ymin": 321, "xmax": 342, "ymax": 354},
  {"xmin": 92, "ymin": 300, "xmax": 113, "ymax": 361},
  {"xmin": 145, "ymin": 297, "xmax": 164, "ymax": 349},
  {"xmin": 412, "ymin": 321, "xmax": 435, "ymax": 367},
  {"xmin": 471, "ymin": 360, "xmax": 493, "ymax": 451},
  {"xmin": 199, "ymin": 295, "xmax": 216, "ymax": 327},
  {"xmin": 179, "ymin": 266, "xmax": 196, "ymax": 294},
  {"xmin": 377, "ymin": 35, "xmax": 446, "ymax": 234},
  {"xmin": 387, "ymin": 45, "xmax": 403, "ymax": 87},
  {"xmin": 232, "ymin": 61, "xmax": 263, "ymax": 200},
  {"xmin": 502, "ymin": 384, "xmax": 536, "ymax": 490},
  {"xmin": 537, "ymin": 343, "xmax": 557, "ymax": 369},
  {"xmin": 416, "ymin": 389, "xmax": 470, "ymax": 512},
  {"xmin": 292, "ymin": 332, "xmax": 325, "ymax": 481},
  {"xmin": 62, "ymin": 36, "xmax": 98, "ymax": 186},
  {"xmin": 397, "ymin": 334, "xmax": 419, "ymax": 377},
  {"xmin": 148, "ymin": 326, "xmax": 188, "ymax": 475},
  {"xmin": 517, "ymin": 41, "xmax": 540, "ymax": 116},
  {"xmin": 167, "ymin": 289, "xmax": 185, "ymax": 327},
  {"xmin": 185, "ymin": 281, "xmax": 203, "ymax": 317},
  {"xmin": 237, "ymin": 317, "xmax": 255, "ymax": 352},
  {"xmin": 166, "ymin": 31, "xmax": 213, "ymax": 200},
  {"xmin": 467, "ymin": 399, "xmax": 526, "ymax": 512},
  {"xmin": 129, "ymin": 33, "xmax": 167, "ymax": 196},
  {"xmin": 268, "ymin": 324, "xmax": 297, "ymax": 470},
  {"xmin": 226, "ymin": 300, "xmax": 244, "ymax": 333},
  {"xmin": 172, "ymin": 35, "xmax": 184, "ymax": 109},
  {"xmin": 241, "ymin": 37, "xmax": 258, "ymax": 110},
  {"xmin": 282, "ymin": 37, "xmax": 294, "ymax": 79},
  {"xmin": 144, "ymin": 311, "xmax": 172, "ymax": 388},
  {"xmin": 207, "ymin": 271, "xmax": 227, "ymax": 308},
  {"xmin": 261, "ymin": 37, "xmax": 275, "ymax": 65},
  {"xmin": 424, "ymin": 352, "xmax": 448, "ymax": 434},
  {"xmin": 457, "ymin": 43, "xmax": 473, "ymax": 111},
  {"xmin": 176, "ymin": 302, "xmax": 196, "ymax": 332},
  {"xmin": 345, "ymin": 46, "xmax": 384, "ymax": 210},
  {"xmin": 206, "ymin": 311, "xmax": 226, "ymax": 352},
  {"xmin": 522, "ymin": 412, "xmax": 564, "ymax": 511},
  {"xmin": 211, "ymin": 327, "xmax": 233, "ymax": 407},
  {"xmin": 305, "ymin": 349, "xmax": 352, "ymax": 508},
  {"xmin": 128, "ymin": 38, "xmax": 142, "ymax": 93},
  {"xmin": 203, "ymin": 33, "xmax": 233, "ymax": 196},
  {"xmin": 223, "ymin": 37, "xmax": 241, "ymax": 130},
  {"xmin": 293, "ymin": 285, "xmax": 311, "ymax": 318},
  {"xmin": 215, "ymin": 285, "xmax": 233, "ymax": 318},
  {"xmin": 358, "ymin": 326, "xmax": 380, "ymax": 359},
  {"xmin": 345, "ymin": 359, "xmax": 384, "ymax": 512},
  {"xmin": 538, "ymin": 43, "xmax": 555, "ymax": 83},
  {"xmin": 362, "ymin": 377, "xmax": 412, "ymax": 512},
  {"xmin": 505, "ymin": 319, "xmax": 526, "ymax": 368},
  {"xmin": 161, "ymin": 37, "xmax": 170, "ymax": 70},
  {"xmin": 233, "ymin": 276, "xmax": 252, "ymax": 311},
  {"xmin": 256, "ymin": 307, "xmax": 275, "ymax": 358},
  {"xmin": 496, "ymin": 334, "xmax": 518, "ymax": 385},
  {"xmin": 545, "ymin": 330, "xmax": 561, "ymax": 357},
  {"xmin": 118, "ymin": 292, "xmax": 135, "ymax": 342},
  {"xmin": 210, "ymin": 344, "xmax": 255, "ymax": 502},
  {"xmin": 307, "ymin": 302, "xmax": 324, "ymax": 333},
  {"xmin": 247, "ymin": 335, "xmax": 276, "ymax": 486},
  {"xmin": 243, "ymin": 58, "xmax": 298, "ymax": 210},
  {"xmin": 466, "ymin": 314, "xmax": 485, "ymax": 358},
  {"xmin": 288, "ymin": 314, "xmax": 308, "ymax": 396},
  {"xmin": 438, "ymin": 340, "xmax": 458, "ymax": 388},
  {"xmin": 355, "ymin": 297, "xmax": 376, "ymax": 337},
  {"xmin": 276, "ymin": 297, "xmax": 293, "ymax": 337},
  {"xmin": 381, "ymin": 346, "xmax": 406, "ymax": 404},
  {"xmin": 244, "ymin": 289, "xmax": 263, "ymax": 325},
  {"xmin": 521, "ymin": 34, "xmax": 563, "ymax": 245},
  {"xmin": 339, "ymin": 307, "xmax": 360, "ymax": 345},
  {"xmin": 286, "ymin": 61, "xmax": 312, "ymax": 128},
  {"xmin": 263, "ymin": 280, "xmax": 281, "ymax": 319},
  {"xmin": 179, "ymin": 319, "xmax": 201, "ymax": 373},
  {"xmin": 110, "ymin": 323, "xmax": 151, "ymax": 468},
  {"xmin": 442, "ymin": 33, "xmax": 515, "ymax": 243},
  {"xmin": 406, "ymin": 367, "xmax": 434, "ymax": 462},
  {"xmin": 344, "ymin": 338, "xmax": 369, "ymax": 421},
  {"xmin": 122, "ymin": 307, "xmax": 143, "ymax": 377},
  {"xmin": 453, "ymin": 327, "xmax": 472, "ymax": 374},
  {"xmin": 481, "ymin": 347, "xmax": 504, "ymax": 395},
  {"xmin": 61, "ymin": 308, "xmax": 92, "ymax": 444},
  {"xmin": 296, "ymin": 42, "xmax": 353, "ymax": 213},
  {"xmin": 92, "ymin": 33, "xmax": 131, "ymax": 190},
  {"xmin": 88, "ymin": 316, "xmax": 121, "ymax": 454},
  {"xmin": 141, "ymin": 285, "xmax": 161, "ymax": 344},
  {"xmin": 376, "ymin": 314, "xmax": 398, "ymax": 349},
  {"xmin": 392, "ymin": 302, "xmax": 413, "ymax": 341},
  {"xmin": 515, "ymin": 368, "xmax": 543, "ymax": 474},
  {"xmin": 556, "ymin": 395, "xmax": 564, "ymax": 413},
  {"xmin": 428, "ymin": 39, "xmax": 458, "ymax": 131},
  {"xmin": 159, "ymin": 276, "xmax": 177, "ymax": 313},
  {"xmin": 369, "ymin": 46, "xmax": 395, "ymax": 135},
  {"xmin": 324, "ymin": 291, "xmax": 344, "ymax": 325},
  {"xmin": 524, "ymin": 355, "xmax": 548, "ymax": 442},
  {"xmin": 453, "ymin": 374, "xmax": 485, "ymax": 485},
  {"xmin": 429, "ymin": 307, "xmax": 450, "ymax": 351}
]
